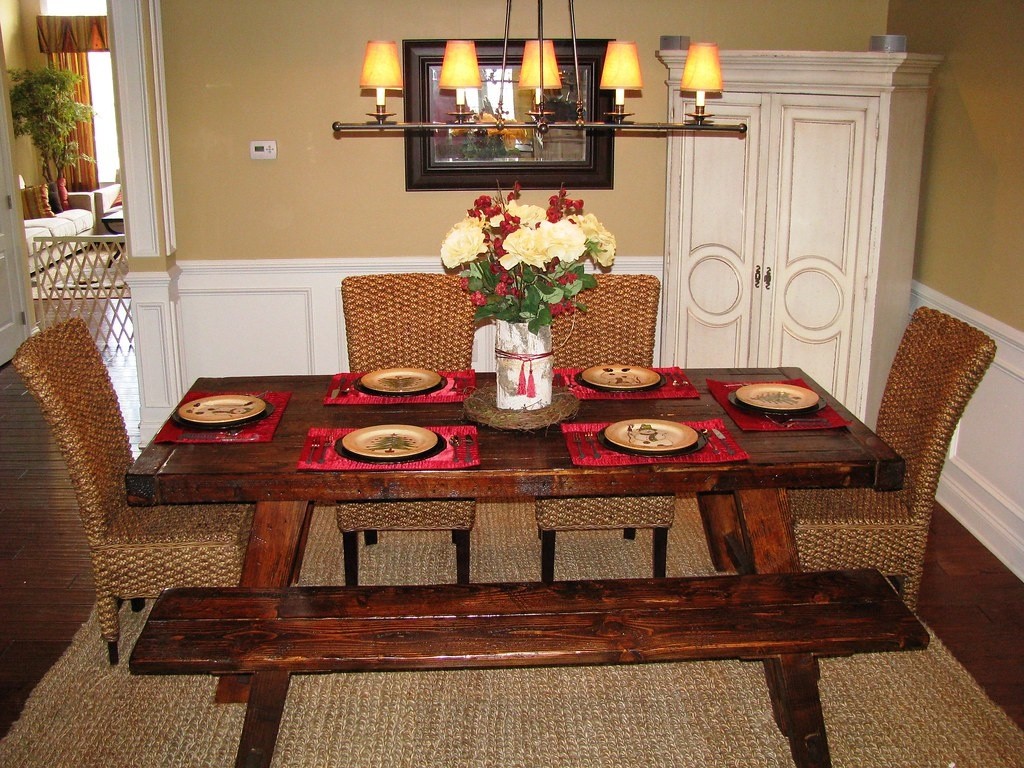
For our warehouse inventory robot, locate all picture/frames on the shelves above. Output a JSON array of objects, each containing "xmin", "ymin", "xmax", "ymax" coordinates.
[{"xmin": 402, "ymin": 39, "xmax": 614, "ymax": 192}]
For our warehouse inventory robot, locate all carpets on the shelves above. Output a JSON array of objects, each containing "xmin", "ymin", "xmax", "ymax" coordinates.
[
  {"xmin": 30, "ymin": 244, "xmax": 132, "ymax": 300},
  {"xmin": 0, "ymin": 503, "xmax": 1024, "ymax": 768}
]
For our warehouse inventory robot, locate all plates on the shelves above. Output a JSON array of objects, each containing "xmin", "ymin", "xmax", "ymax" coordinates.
[
  {"xmin": 603, "ymin": 419, "xmax": 699, "ymax": 451},
  {"xmin": 179, "ymin": 396, "xmax": 266, "ymax": 423},
  {"xmin": 342, "ymin": 424, "xmax": 438, "ymax": 458},
  {"xmin": 581, "ymin": 365, "xmax": 661, "ymax": 390},
  {"xmin": 736, "ymin": 383, "xmax": 818, "ymax": 409},
  {"xmin": 360, "ymin": 367, "xmax": 442, "ymax": 392}
]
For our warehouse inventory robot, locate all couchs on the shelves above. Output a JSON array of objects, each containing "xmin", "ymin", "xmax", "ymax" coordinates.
[
  {"xmin": 95, "ymin": 167, "xmax": 125, "ymax": 235},
  {"xmin": 23, "ymin": 192, "xmax": 97, "ymax": 274}
]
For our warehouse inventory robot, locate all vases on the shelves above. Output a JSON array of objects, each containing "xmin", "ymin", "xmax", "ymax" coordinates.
[{"xmin": 494, "ymin": 316, "xmax": 551, "ymax": 412}]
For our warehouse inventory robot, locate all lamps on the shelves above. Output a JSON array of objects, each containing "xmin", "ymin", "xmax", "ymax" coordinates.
[{"xmin": 332, "ymin": 0, "xmax": 748, "ymax": 133}]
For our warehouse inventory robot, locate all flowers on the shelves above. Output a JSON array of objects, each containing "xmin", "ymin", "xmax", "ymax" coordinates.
[
  {"xmin": 449, "ymin": 108, "xmax": 529, "ymax": 161},
  {"xmin": 441, "ymin": 177, "xmax": 616, "ymax": 336}
]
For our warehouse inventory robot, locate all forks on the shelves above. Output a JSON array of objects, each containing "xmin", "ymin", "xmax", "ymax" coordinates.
[
  {"xmin": 585, "ymin": 429, "xmax": 601, "ymax": 458},
  {"xmin": 573, "ymin": 432, "xmax": 584, "ymax": 458},
  {"xmin": 307, "ymin": 433, "xmax": 333, "ymax": 464},
  {"xmin": 765, "ymin": 415, "xmax": 828, "ymax": 427}
]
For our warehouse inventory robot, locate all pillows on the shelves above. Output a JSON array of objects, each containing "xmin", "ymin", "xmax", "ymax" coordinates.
[{"xmin": 22, "ymin": 178, "xmax": 72, "ymax": 220}]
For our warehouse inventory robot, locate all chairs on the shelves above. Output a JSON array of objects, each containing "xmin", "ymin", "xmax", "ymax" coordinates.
[
  {"xmin": 337, "ymin": 273, "xmax": 477, "ymax": 588},
  {"xmin": 785, "ymin": 305, "xmax": 998, "ymax": 618},
  {"xmin": 12, "ymin": 317, "xmax": 257, "ymax": 667},
  {"xmin": 534, "ymin": 274, "xmax": 674, "ymax": 579}
]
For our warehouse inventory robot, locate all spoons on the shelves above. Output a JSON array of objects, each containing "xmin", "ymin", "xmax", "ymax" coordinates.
[
  {"xmin": 449, "ymin": 435, "xmax": 463, "ymax": 462},
  {"xmin": 701, "ymin": 428, "xmax": 721, "ymax": 455}
]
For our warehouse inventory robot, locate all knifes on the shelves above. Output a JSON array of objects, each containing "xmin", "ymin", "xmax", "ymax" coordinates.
[
  {"xmin": 330, "ymin": 377, "xmax": 346, "ymax": 399},
  {"xmin": 712, "ymin": 428, "xmax": 733, "ymax": 455},
  {"xmin": 464, "ymin": 435, "xmax": 474, "ymax": 462}
]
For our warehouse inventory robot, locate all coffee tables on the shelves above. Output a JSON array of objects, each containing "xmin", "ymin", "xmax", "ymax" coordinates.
[{"xmin": 101, "ymin": 209, "xmax": 126, "ymax": 268}]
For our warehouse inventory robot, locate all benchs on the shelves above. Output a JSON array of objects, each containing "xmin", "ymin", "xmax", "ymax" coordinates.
[{"xmin": 128, "ymin": 569, "xmax": 931, "ymax": 768}]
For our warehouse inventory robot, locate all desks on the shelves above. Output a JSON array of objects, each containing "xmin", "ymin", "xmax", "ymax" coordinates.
[{"xmin": 125, "ymin": 368, "xmax": 903, "ymax": 736}]
